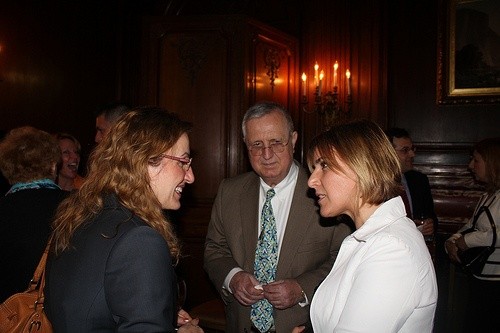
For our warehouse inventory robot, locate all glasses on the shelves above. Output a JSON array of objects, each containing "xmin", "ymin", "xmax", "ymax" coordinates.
[
  {"xmin": 161, "ymin": 154, "xmax": 193, "ymax": 171},
  {"xmin": 246, "ymin": 135, "xmax": 291, "ymax": 156}
]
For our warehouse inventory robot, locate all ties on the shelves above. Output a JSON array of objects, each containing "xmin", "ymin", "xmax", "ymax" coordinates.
[{"xmin": 250, "ymin": 188, "xmax": 279, "ymax": 333}]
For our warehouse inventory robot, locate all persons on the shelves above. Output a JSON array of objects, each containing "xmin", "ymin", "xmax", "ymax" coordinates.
[
  {"xmin": 94, "ymin": 105, "xmax": 135, "ymax": 145},
  {"xmin": 384, "ymin": 126, "xmax": 438, "ymax": 258},
  {"xmin": 441, "ymin": 138, "xmax": 500, "ymax": 333},
  {"xmin": 201, "ymin": 102, "xmax": 356, "ymax": 333},
  {"xmin": 50, "ymin": 132, "xmax": 85, "ymax": 192},
  {"xmin": 40, "ymin": 107, "xmax": 207, "ymax": 332},
  {"xmin": 0, "ymin": 124, "xmax": 75, "ymax": 303},
  {"xmin": 305, "ymin": 109, "xmax": 439, "ymax": 333}
]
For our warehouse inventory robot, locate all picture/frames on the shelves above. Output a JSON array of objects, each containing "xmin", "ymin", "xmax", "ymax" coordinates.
[{"xmin": 436, "ymin": 0, "xmax": 500, "ymax": 105}]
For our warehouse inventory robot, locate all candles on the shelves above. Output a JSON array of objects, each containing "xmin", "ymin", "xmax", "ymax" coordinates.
[
  {"xmin": 314, "ymin": 63, "xmax": 319, "ymax": 88},
  {"xmin": 345, "ymin": 69, "xmax": 351, "ymax": 94},
  {"xmin": 333, "ymin": 60, "xmax": 338, "ymax": 86},
  {"xmin": 318, "ymin": 70, "xmax": 325, "ymax": 96},
  {"xmin": 302, "ymin": 72, "xmax": 307, "ymax": 96}
]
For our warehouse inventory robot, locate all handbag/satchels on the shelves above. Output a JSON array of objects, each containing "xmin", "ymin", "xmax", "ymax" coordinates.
[
  {"xmin": 456, "ymin": 206, "xmax": 496, "ymax": 277},
  {"xmin": 0, "ymin": 286, "xmax": 54, "ymax": 333}
]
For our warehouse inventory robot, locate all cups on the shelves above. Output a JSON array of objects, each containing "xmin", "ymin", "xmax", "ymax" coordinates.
[{"xmin": 415, "ymin": 213, "xmax": 426, "ymax": 227}]
